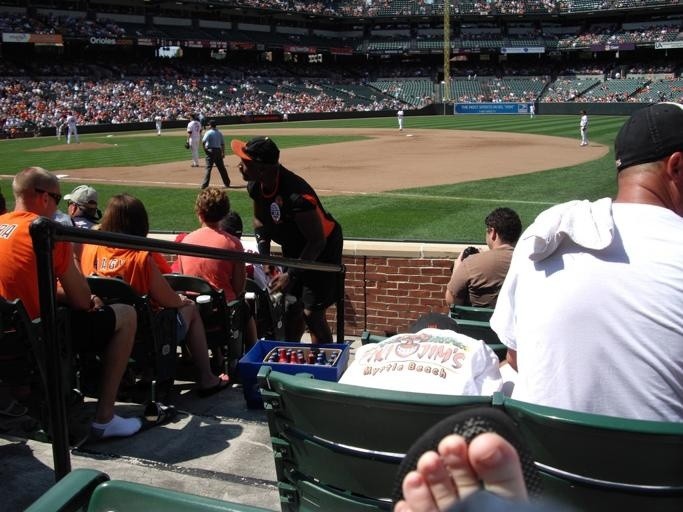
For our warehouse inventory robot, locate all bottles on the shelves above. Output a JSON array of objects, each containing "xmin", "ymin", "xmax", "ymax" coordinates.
[{"xmin": 268, "ymin": 346, "xmax": 338, "ymax": 366}]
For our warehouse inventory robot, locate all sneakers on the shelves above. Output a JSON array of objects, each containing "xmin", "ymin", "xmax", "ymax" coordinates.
[{"xmin": 62, "ymin": 185, "xmax": 99, "ymax": 208}]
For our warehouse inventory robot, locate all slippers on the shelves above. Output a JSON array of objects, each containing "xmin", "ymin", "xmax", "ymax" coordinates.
[
  {"xmin": 0, "ymin": 397, "xmax": 30, "ymax": 417},
  {"xmin": 198, "ymin": 372, "xmax": 231, "ymax": 398},
  {"xmin": 138, "ymin": 399, "xmax": 178, "ymax": 427}
]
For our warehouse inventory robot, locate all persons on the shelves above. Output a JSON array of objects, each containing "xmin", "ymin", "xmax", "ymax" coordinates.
[
  {"xmin": 228, "ymin": 136, "xmax": 345, "ymax": 344},
  {"xmin": 332, "ymin": 311, "xmax": 505, "ymax": 502},
  {"xmin": 490, "ymin": 100, "xmax": 683, "ymax": 489},
  {"xmin": 201, "ymin": 119, "xmax": 230, "ymax": 189},
  {"xmin": 186, "ymin": 113, "xmax": 202, "ymax": 167},
  {"xmin": 445, "ymin": 206, "xmax": 522, "ymax": 309}
]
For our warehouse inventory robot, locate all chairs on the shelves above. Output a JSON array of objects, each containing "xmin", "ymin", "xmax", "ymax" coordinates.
[
  {"xmin": 361, "ymin": 304, "xmax": 508, "ymax": 364},
  {"xmin": 23, "ymin": 468, "xmax": 275, "ymax": 511},
  {"xmin": 491, "ymin": 393, "xmax": 683, "ymax": 512},
  {"xmin": 0, "ymin": 273, "xmax": 283, "ymax": 440},
  {"xmin": 256, "ymin": 366, "xmax": 503, "ymax": 511},
  {"xmin": 0, "ymin": 0, "xmax": 683, "ymax": 105}
]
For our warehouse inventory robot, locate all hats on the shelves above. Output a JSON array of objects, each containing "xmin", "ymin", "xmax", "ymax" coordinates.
[
  {"xmin": 613, "ymin": 101, "xmax": 683, "ymax": 173},
  {"xmin": 229, "ymin": 136, "xmax": 281, "ymax": 165}
]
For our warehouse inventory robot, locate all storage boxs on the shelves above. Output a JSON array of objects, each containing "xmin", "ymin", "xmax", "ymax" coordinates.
[
  {"xmin": 263, "ymin": 347, "xmax": 342, "ymax": 368},
  {"xmin": 240, "ymin": 340, "xmax": 350, "ymax": 411}
]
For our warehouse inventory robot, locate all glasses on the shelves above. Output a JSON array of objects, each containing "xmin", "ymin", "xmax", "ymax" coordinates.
[{"xmin": 31, "ymin": 187, "xmax": 61, "ymax": 205}]
